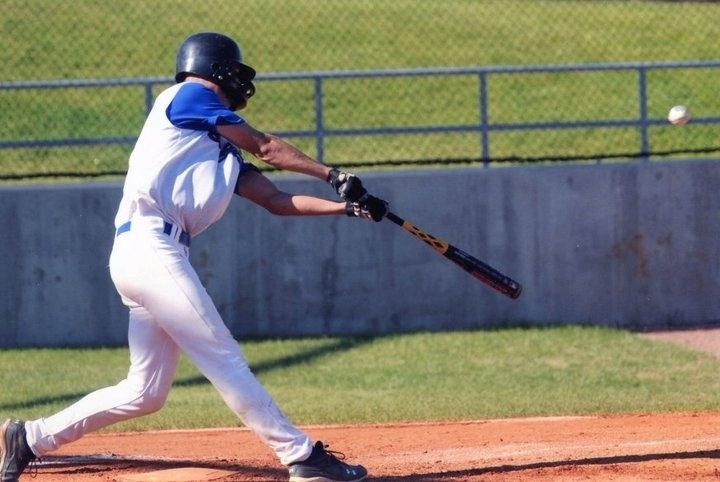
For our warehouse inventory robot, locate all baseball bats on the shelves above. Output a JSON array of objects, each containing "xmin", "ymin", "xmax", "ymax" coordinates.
[{"xmin": 339, "ymin": 182, "xmax": 521, "ymax": 300}]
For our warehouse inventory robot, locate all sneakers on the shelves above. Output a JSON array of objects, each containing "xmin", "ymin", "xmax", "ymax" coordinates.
[
  {"xmin": 0, "ymin": 419, "xmax": 36, "ymax": 482},
  {"xmin": 288, "ymin": 440, "xmax": 367, "ymax": 482}
]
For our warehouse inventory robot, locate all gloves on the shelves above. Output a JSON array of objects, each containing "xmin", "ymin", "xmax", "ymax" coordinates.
[
  {"xmin": 346, "ymin": 193, "xmax": 388, "ymax": 222},
  {"xmin": 326, "ymin": 168, "xmax": 363, "ymax": 201}
]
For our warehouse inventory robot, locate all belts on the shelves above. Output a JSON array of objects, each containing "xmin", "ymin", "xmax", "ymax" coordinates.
[{"xmin": 116, "ymin": 217, "xmax": 190, "ymax": 247}]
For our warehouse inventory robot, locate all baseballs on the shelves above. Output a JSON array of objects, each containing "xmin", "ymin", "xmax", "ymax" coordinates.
[{"xmin": 666, "ymin": 106, "xmax": 691, "ymax": 128}]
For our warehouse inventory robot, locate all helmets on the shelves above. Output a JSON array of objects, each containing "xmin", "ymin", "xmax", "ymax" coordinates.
[{"xmin": 175, "ymin": 32, "xmax": 257, "ymax": 112}]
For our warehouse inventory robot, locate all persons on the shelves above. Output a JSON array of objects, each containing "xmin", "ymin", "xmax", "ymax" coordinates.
[{"xmin": 1, "ymin": 30, "xmax": 390, "ymax": 482}]
